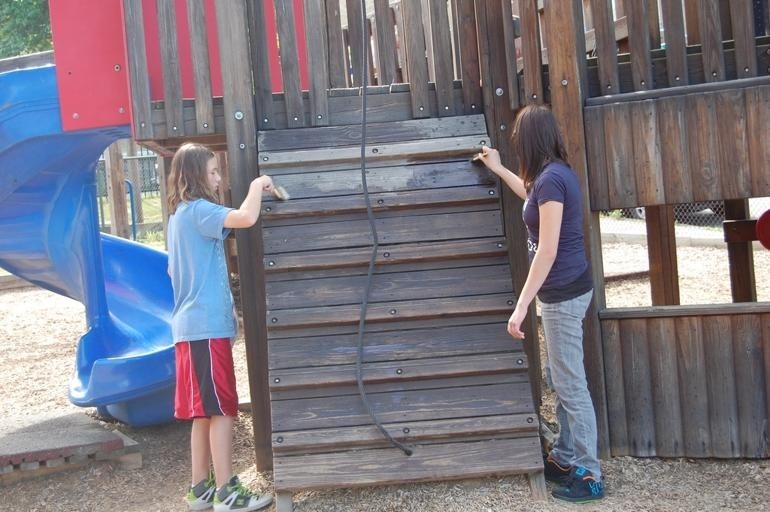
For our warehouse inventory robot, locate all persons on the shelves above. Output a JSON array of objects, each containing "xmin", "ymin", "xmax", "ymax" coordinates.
[
  {"xmin": 478, "ymin": 106, "xmax": 604, "ymax": 501},
  {"xmin": 166, "ymin": 142, "xmax": 274, "ymax": 512}
]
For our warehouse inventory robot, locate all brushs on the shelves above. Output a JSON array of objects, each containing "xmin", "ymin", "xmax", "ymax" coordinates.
[
  {"xmin": 471, "ymin": 153, "xmax": 490, "ymax": 163},
  {"xmin": 273, "ymin": 186, "xmax": 290, "ymax": 200}
]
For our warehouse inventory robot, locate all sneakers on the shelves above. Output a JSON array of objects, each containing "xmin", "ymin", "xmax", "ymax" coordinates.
[
  {"xmin": 213, "ymin": 475, "xmax": 273, "ymax": 512},
  {"xmin": 184, "ymin": 471, "xmax": 215, "ymax": 510},
  {"xmin": 551, "ymin": 467, "xmax": 603, "ymax": 504},
  {"xmin": 542, "ymin": 457, "xmax": 573, "ymax": 482}
]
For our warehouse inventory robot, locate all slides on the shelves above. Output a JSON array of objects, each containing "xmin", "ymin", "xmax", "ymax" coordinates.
[{"xmin": 0, "ymin": 64, "xmax": 238, "ymax": 426}]
